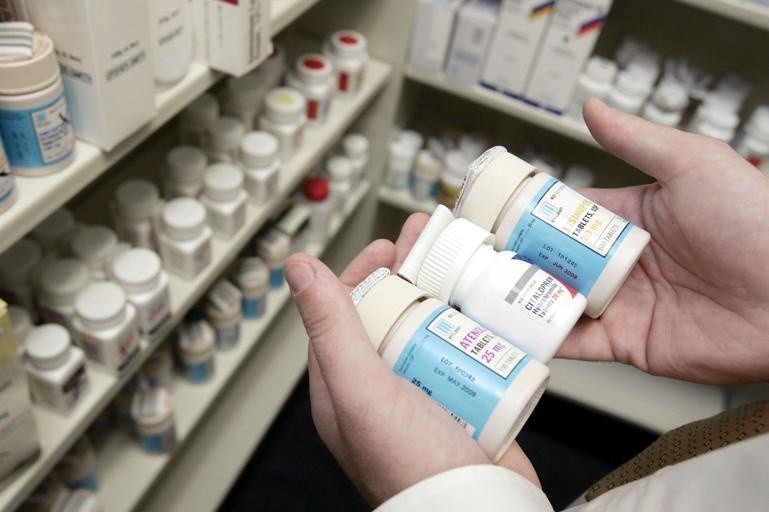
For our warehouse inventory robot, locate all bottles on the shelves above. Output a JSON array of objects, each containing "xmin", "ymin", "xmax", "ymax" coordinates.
[
  {"xmin": 3, "ymin": 28, "xmax": 367, "ymax": 501},
  {"xmin": 353, "ymin": 269, "xmax": 554, "ymax": 466},
  {"xmin": 397, "ymin": 206, "xmax": 587, "ymax": 365},
  {"xmin": 453, "ymin": 144, "xmax": 652, "ymax": 322},
  {"xmin": 571, "ymin": 39, "xmax": 768, "ymax": 166},
  {"xmin": 383, "ymin": 123, "xmax": 598, "ymax": 215}
]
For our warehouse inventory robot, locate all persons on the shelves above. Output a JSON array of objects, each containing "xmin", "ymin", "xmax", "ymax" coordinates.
[{"xmin": 280, "ymin": 92, "xmax": 768, "ymax": 512}]
